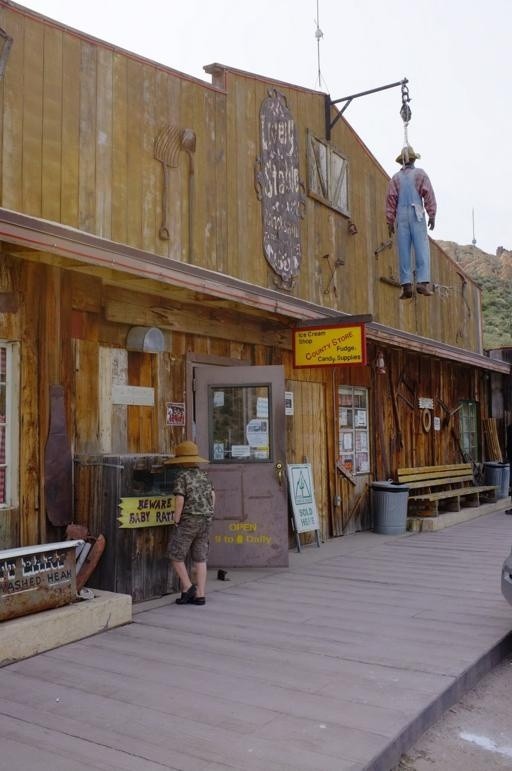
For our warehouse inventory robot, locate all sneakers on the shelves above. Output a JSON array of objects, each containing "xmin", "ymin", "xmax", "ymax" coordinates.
[{"xmin": 176, "ymin": 584, "xmax": 205, "ymax": 605}]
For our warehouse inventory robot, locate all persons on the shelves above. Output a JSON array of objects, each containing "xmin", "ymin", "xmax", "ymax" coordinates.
[
  {"xmin": 386, "ymin": 147, "xmax": 437, "ymax": 299},
  {"xmin": 163, "ymin": 440, "xmax": 216, "ymax": 605}
]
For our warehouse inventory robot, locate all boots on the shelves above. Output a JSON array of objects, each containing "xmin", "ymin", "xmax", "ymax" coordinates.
[
  {"xmin": 416, "ymin": 285, "xmax": 433, "ymax": 296},
  {"xmin": 398, "ymin": 284, "xmax": 412, "ymax": 299}
]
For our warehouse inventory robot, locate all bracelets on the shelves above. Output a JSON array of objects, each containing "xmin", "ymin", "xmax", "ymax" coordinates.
[{"xmin": 173, "ymin": 519, "xmax": 180, "ymax": 526}]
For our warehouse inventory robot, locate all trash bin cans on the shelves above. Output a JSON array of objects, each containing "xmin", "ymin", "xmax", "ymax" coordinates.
[
  {"xmin": 371, "ymin": 481, "xmax": 409, "ymax": 535},
  {"xmin": 482, "ymin": 462, "xmax": 510, "ymax": 499}
]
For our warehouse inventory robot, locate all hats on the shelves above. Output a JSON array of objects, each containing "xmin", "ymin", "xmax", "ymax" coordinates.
[
  {"xmin": 164, "ymin": 440, "xmax": 210, "ymax": 464},
  {"xmin": 395, "ymin": 146, "xmax": 420, "ymax": 162}
]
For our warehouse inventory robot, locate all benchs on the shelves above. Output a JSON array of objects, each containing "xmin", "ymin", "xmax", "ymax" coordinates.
[{"xmin": 397, "ymin": 463, "xmax": 502, "ymax": 517}]
